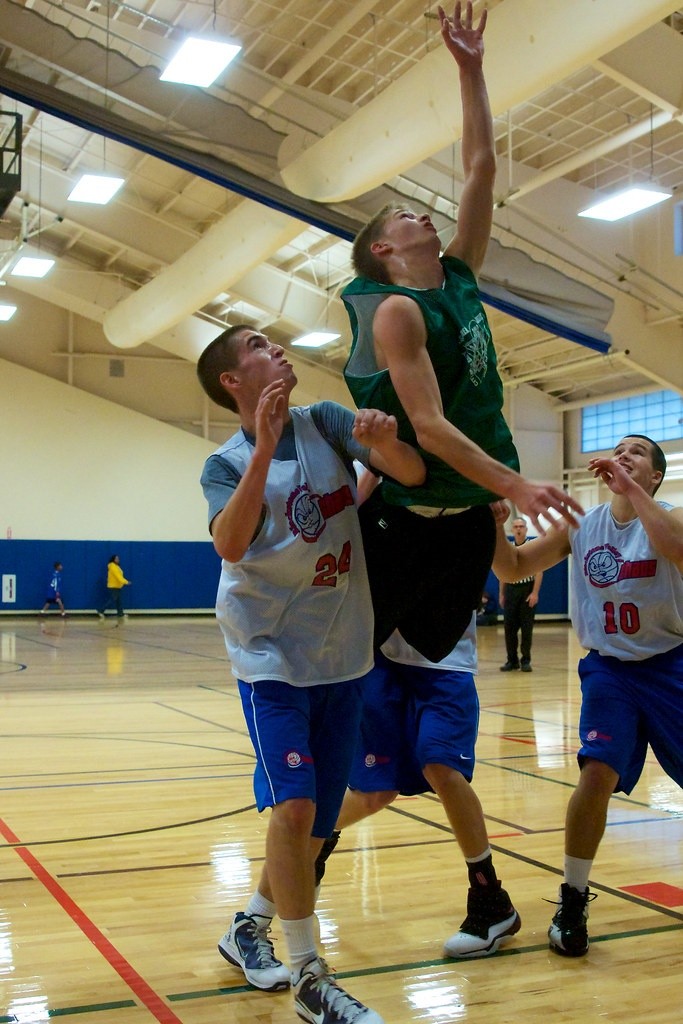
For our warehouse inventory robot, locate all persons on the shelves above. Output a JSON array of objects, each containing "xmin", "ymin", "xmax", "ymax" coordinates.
[
  {"xmin": 196, "ymin": 324, "xmax": 427, "ymax": 1024},
  {"xmin": 497, "ymin": 517, "xmax": 544, "ymax": 673},
  {"xmin": 476, "ymin": 594, "xmax": 497, "ymax": 625},
  {"xmin": 39, "ymin": 562, "xmax": 69, "ymax": 618},
  {"xmin": 96, "ymin": 554, "xmax": 131, "ymax": 618},
  {"xmin": 312, "ymin": 470, "xmax": 523, "ymax": 960},
  {"xmin": 490, "ymin": 433, "xmax": 683, "ymax": 957},
  {"xmin": 340, "ymin": 0, "xmax": 521, "ymax": 661}
]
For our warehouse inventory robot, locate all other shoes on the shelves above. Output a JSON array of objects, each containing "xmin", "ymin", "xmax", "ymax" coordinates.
[
  {"xmin": 522, "ymin": 664, "xmax": 532, "ymax": 671},
  {"xmin": 500, "ymin": 661, "xmax": 520, "ymax": 671}
]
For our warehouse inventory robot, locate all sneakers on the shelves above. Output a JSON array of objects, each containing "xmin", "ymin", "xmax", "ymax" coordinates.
[
  {"xmin": 443, "ymin": 879, "xmax": 522, "ymax": 960},
  {"xmin": 218, "ymin": 911, "xmax": 292, "ymax": 992},
  {"xmin": 542, "ymin": 883, "xmax": 598, "ymax": 957},
  {"xmin": 291, "ymin": 958, "xmax": 384, "ymax": 1024}
]
